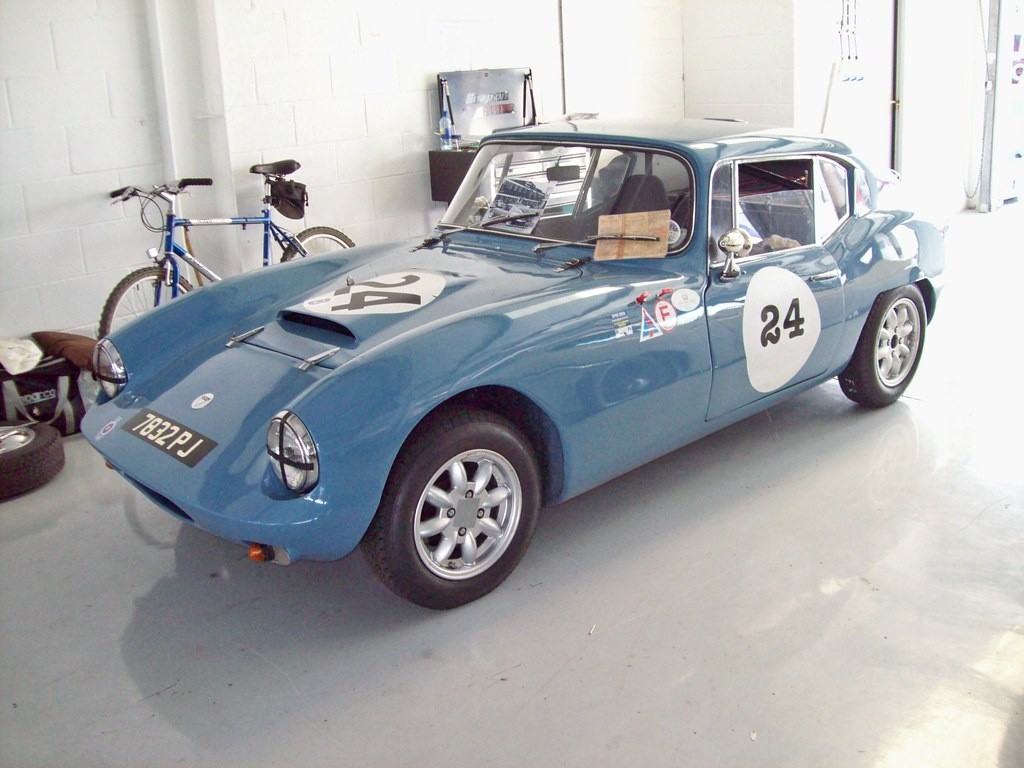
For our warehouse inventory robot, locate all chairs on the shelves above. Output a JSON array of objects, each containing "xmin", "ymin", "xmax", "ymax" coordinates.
[{"xmin": 610, "ymin": 174, "xmax": 668, "ymax": 233}]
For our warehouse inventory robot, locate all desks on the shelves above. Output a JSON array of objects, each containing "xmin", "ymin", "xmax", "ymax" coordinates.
[{"xmin": 429, "ymin": 147, "xmax": 478, "ymax": 208}]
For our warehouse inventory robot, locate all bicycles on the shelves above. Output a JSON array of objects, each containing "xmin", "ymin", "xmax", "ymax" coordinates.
[{"xmin": 96, "ymin": 160, "xmax": 357, "ymax": 341}]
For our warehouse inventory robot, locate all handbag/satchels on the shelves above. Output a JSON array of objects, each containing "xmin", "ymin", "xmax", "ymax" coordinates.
[{"xmin": 0, "ymin": 352, "xmax": 85, "ymax": 437}]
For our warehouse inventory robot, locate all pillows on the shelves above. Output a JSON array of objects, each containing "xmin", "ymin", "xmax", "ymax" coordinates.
[{"xmin": 32, "ymin": 329, "xmax": 96, "ymax": 368}]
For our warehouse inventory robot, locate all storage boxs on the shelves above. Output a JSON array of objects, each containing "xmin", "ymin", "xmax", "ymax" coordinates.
[{"xmin": 0, "ymin": 352, "xmax": 86, "ymax": 437}]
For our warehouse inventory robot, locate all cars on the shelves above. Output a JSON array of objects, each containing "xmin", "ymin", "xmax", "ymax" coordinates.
[{"xmin": 79, "ymin": 111, "xmax": 950, "ymax": 611}]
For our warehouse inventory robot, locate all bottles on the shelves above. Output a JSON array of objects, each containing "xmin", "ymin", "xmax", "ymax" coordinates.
[{"xmin": 439, "ymin": 111, "xmax": 452, "ymax": 150}]
